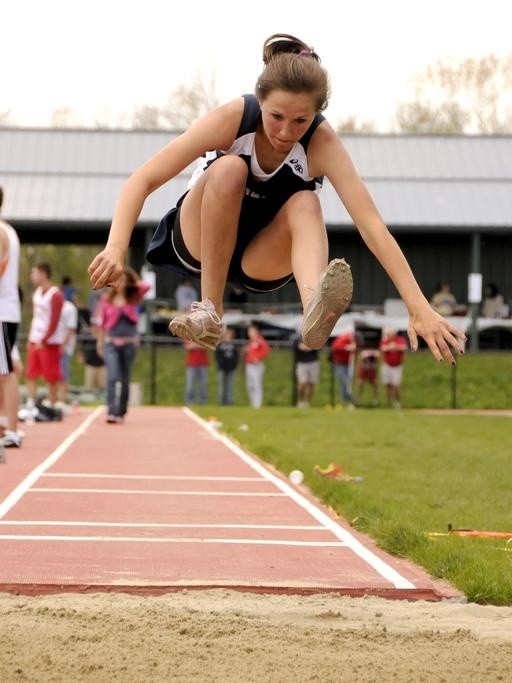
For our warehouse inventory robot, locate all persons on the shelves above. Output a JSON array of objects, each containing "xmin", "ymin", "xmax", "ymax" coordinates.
[
  {"xmin": 182, "ymin": 339, "xmax": 210, "ymax": 406},
  {"xmin": 358, "ymin": 326, "xmax": 409, "ymax": 411},
  {"xmin": 243, "ymin": 325, "xmax": 270, "ymax": 409},
  {"xmin": 330, "ymin": 329, "xmax": 356, "ymax": 407},
  {"xmin": 18, "ymin": 262, "xmax": 88, "ymax": 422},
  {"xmin": 0, "ymin": 188, "xmax": 24, "ymax": 448},
  {"xmin": 430, "ymin": 281, "xmax": 456, "ymax": 316},
  {"xmin": 296, "ymin": 336, "xmax": 321, "ymax": 410},
  {"xmin": 174, "ymin": 279, "xmax": 199, "ymax": 312},
  {"xmin": 214, "ymin": 327, "xmax": 237, "ymax": 406},
  {"xmin": 84, "ymin": 265, "xmax": 149, "ymax": 424},
  {"xmin": 88, "ymin": 34, "xmax": 468, "ymax": 366}
]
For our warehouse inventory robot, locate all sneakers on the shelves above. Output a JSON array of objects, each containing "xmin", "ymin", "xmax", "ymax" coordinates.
[
  {"xmin": 168, "ymin": 299, "xmax": 225, "ymax": 352},
  {"xmin": 302, "ymin": 258, "xmax": 354, "ymax": 351}
]
